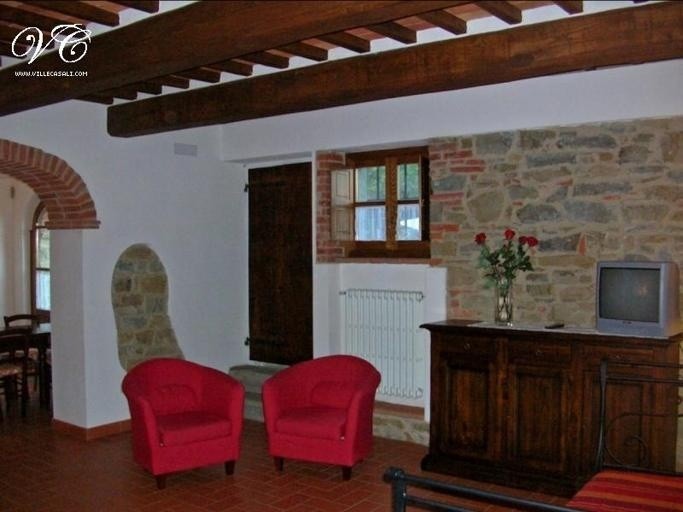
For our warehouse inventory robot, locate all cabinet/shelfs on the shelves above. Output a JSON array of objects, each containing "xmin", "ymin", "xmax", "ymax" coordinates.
[{"xmin": 420, "ymin": 320, "xmax": 683, "ymax": 500}]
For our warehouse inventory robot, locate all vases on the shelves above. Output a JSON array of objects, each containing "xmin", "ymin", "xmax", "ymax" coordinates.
[{"xmin": 494, "ymin": 284, "xmax": 513, "ymax": 323}]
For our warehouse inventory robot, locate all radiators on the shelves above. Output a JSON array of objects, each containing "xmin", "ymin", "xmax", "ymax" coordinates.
[{"xmin": 339, "ymin": 288, "xmax": 424, "ymax": 400}]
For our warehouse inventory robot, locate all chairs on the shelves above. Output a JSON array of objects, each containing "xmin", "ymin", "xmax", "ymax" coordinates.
[
  {"xmin": 260, "ymin": 353, "xmax": 382, "ymax": 481},
  {"xmin": 0, "ymin": 314, "xmax": 52, "ymax": 419},
  {"xmin": 121, "ymin": 357, "xmax": 247, "ymax": 490}
]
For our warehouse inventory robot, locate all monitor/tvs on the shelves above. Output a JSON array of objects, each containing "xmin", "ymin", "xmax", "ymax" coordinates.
[{"xmin": 596, "ymin": 261, "xmax": 683, "ymax": 339}]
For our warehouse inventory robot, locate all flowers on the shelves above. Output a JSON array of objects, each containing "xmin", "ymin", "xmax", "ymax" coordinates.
[{"xmin": 473, "ymin": 227, "xmax": 539, "ymax": 314}]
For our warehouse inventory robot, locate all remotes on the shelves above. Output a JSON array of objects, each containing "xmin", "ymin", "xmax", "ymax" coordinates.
[{"xmin": 544, "ymin": 323, "xmax": 565, "ymax": 328}]
